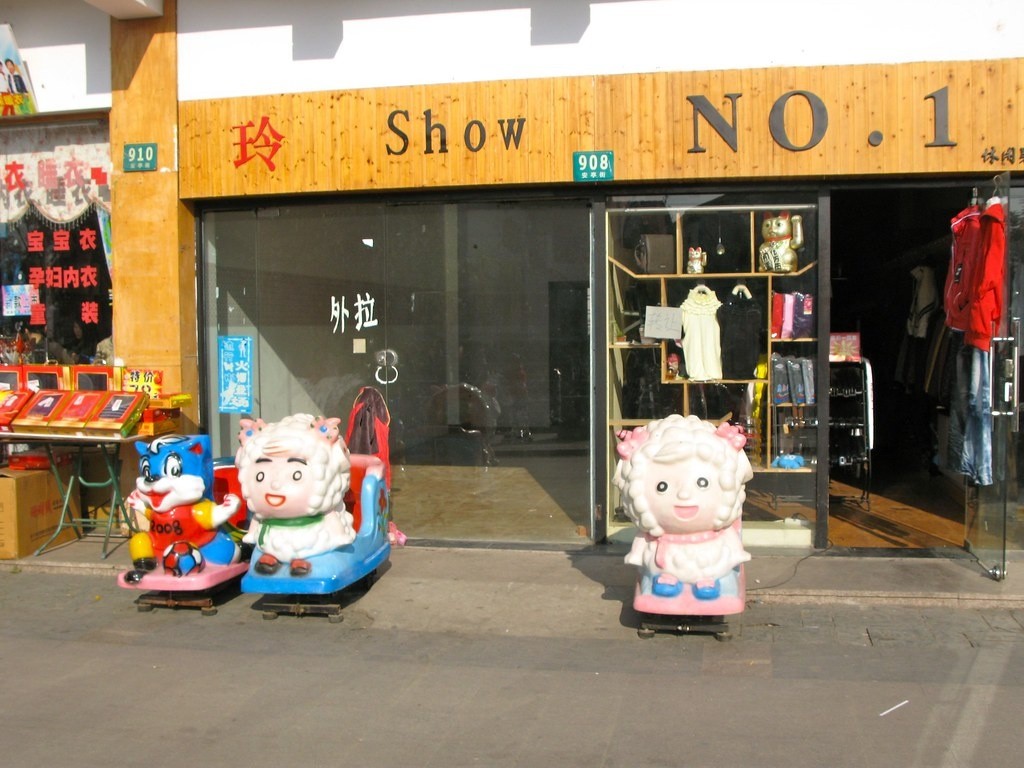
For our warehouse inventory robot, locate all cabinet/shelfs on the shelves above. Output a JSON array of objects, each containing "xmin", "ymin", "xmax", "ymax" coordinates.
[{"xmin": 605, "ymin": 204, "xmax": 817, "ymax": 473}]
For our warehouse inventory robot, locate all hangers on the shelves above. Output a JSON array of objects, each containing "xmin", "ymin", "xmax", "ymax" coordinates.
[
  {"xmin": 731, "ymin": 279, "xmax": 753, "ymax": 300},
  {"xmin": 969, "ymin": 174, "xmax": 1003, "ymax": 205},
  {"xmin": 691, "ymin": 279, "xmax": 711, "ymax": 294}
]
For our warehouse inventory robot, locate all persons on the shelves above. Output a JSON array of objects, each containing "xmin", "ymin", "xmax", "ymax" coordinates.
[
  {"xmin": 66, "ymin": 318, "xmax": 94, "ymax": 355},
  {"xmin": 6, "ymin": 60, "xmax": 25, "ymax": 93},
  {"xmin": 0, "ymin": 61, "xmax": 12, "ymax": 93},
  {"xmin": 26, "ymin": 319, "xmax": 75, "ymax": 364}
]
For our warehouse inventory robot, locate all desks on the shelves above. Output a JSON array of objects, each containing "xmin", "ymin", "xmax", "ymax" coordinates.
[{"xmin": 0, "ymin": 434, "xmax": 140, "ymax": 559}]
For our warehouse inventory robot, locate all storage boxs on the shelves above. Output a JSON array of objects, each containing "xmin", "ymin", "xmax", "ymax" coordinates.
[
  {"xmin": 0, "ymin": 364, "xmax": 193, "ymax": 439},
  {"xmin": 0, "ymin": 466, "xmax": 83, "ymax": 559},
  {"xmin": 36, "ymin": 446, "xmax": 119, "ymax": 530}
]
